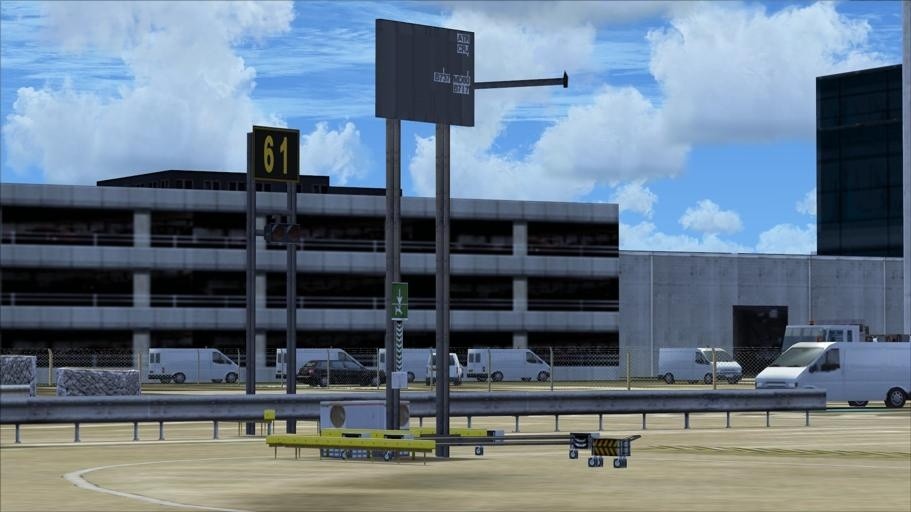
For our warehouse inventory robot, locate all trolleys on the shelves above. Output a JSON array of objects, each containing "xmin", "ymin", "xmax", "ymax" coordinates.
[{"xmin": 587, "ymin": 434, "xmax": 642, "ymax": 468}]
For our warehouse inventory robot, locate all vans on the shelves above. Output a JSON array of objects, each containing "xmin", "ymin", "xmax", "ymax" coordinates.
[
  {"xmin": 657, "ymin": 347, "xmax": 744, "ymax": 385},
  {"xmin": 754, "ymin": 341, "xmax": 911, "ymax": 409},
  {"xmin": 275, "ymin": 348, "xmax": 366, "ymax": 380},
  {"xmin": 378, "ymin": 348, "xmax": 551, "ymax": 385},
  {"xmin": 147, "ymin": 347, "xmax": 240, "ymax": 384}
]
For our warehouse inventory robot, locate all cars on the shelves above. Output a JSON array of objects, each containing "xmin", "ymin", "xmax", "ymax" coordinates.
[{"xmin": 296, "ymin": 359, "xmax": 386, "ymax": 388}]
